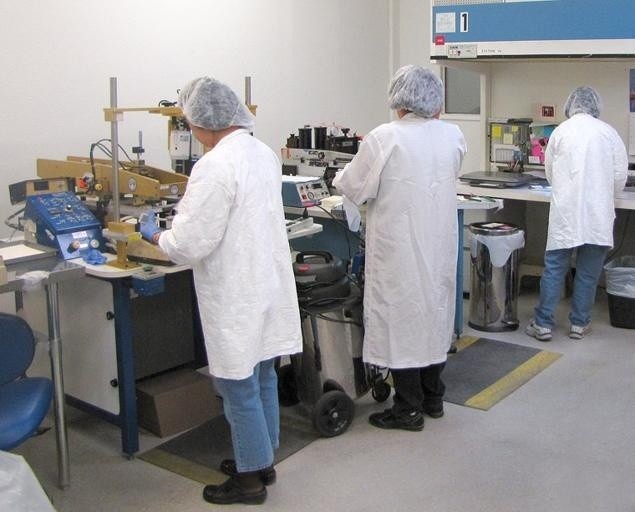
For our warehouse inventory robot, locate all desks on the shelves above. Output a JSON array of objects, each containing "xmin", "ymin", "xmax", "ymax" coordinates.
[
  {"xmin": 0, "ymin": 255, "xmax": 89, "ymax": 492},
  {"xmin": 454, "ymin": 178, "xmax": 635, "ymax": 212}
]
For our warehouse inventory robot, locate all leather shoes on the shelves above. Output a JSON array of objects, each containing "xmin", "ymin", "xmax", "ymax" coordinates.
[
  {"xmin": 202, "ymin": 475, "xmax": 269, "ymax": 508},
  {"xmin": 219, "ymin": 457, "xmax": 280, "ymax": 486}
]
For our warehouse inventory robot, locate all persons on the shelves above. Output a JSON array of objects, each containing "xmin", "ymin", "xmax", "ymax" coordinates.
[
  {"xmin": 525, "ymin": 85, "xmax": 628, "ymax": 341},
  {"xmin": 332, "ymin": 63, "xmax": 468, "ymax": 431},
  {"xmin": 140, "ymin": 73, "xmax": 304, "ymax": 506}
]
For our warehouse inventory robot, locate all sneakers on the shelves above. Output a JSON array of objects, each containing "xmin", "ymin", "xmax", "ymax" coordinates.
[
  {"xmin": 367, "ymin": 406, "xmax": 426, "ymax": 432},
  {"xmin": 524, "ymin": 318, "xmax": 554, "ymax": 342},
  {"xmin": 392, "ymin": 392, "xmax": 445, "ymax": 419},
  {"xmin": 567, "ymin": 320, "xmax": 594, "ymax": 341}
]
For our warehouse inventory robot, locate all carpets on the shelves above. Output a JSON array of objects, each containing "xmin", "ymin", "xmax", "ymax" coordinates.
[
  {"xmin": 379, "ymin": 331, "xmax": 565, "ymax": 414},
  {"xmin": 138, "ymin": 410, "xmax": 320, "ymax": 486}
]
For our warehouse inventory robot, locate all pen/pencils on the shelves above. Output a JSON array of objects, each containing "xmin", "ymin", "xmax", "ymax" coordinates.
[{"xmin": 506, "ymin": 160, "xmax": 519, "ymax": 169}]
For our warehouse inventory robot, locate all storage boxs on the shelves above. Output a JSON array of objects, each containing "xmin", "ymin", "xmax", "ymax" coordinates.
[{"xmin": 136, "ymin": 366, "xmax": 222, "ymax": 439}]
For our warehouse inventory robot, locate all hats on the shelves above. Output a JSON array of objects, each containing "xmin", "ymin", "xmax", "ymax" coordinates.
[
  {"xmin": 178, "ymin": 74, "xmax": 260, "ymax": 129},
  {"xmin": 564, "ymin": 84, "xmax": 604, "ymax": 121},
  {"xmin": 385, "ymin": 61, "xmax": 448, "ymax": 120}
]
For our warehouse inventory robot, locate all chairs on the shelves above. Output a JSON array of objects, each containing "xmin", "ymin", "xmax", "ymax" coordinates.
[{"xmin": 0, "ymin": 308, "xmax": 56, "ymax": 451}]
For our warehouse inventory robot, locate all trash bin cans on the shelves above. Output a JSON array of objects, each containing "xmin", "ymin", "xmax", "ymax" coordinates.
[
  {"xmin": 468, "ymin": 221, "xmax": 521, "ymax": 332},
  {"xmin": 603, "ymin": 256, "xmax": 634, "ymax": 329}
]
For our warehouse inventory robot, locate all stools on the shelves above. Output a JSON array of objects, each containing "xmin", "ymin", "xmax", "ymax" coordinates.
[{"xmin": 517, "ymin": 256, "xmax": 574, "ymax": 301}]
